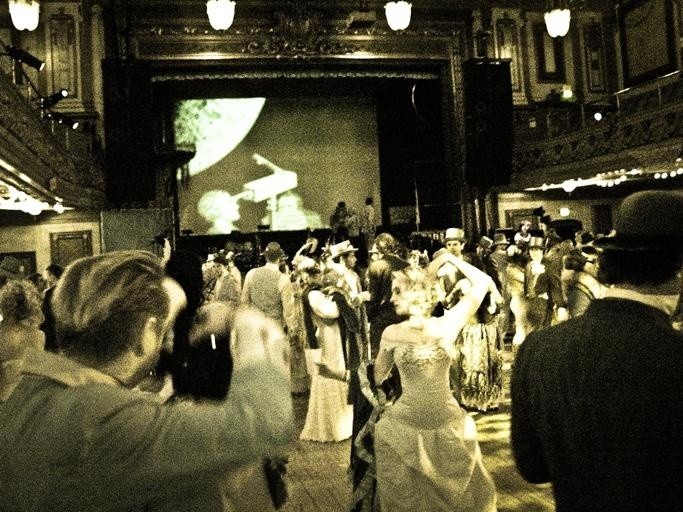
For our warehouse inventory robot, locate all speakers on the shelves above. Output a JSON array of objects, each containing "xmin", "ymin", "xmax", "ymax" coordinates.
[
  {"xmin": 462, "ymin": 58, "xmax": 514, "ymax": 192},
  {"xmin": 103, "ymin": 58, "xmax": 155, "ymax": 202}
]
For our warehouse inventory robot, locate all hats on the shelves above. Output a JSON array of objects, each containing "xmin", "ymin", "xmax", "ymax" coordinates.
[
  {"xmin": 476, "ymin": 236, "xmax": 494, "ymax": 251},
  {"xmin": 527, "ymin": 236, "xmax": 546, "ymax": 251},
  {"xmin": 441, "ymin": 228, "xmax": 467, "ymax": 245},
  {"xmin": 593, "ymin": 190, "xmax": 683, "ymax": 254},
  {"xmin": 368, "ymin": 243, "xmax": 380, "ymax": 253},
  {"xmin": 492, "ymin": 233, "xmax": 511, "ymax": 248},
  {"xmin": 260, "ymin": 242, "xmax": 286, "ymax": 257},
  {"xmin": 329, "ymin": 240, "xmax": 359, "ymax": 259},
  {"xmin": 1, "ymin": 256, "xmax": 25, "ymax": 280}
]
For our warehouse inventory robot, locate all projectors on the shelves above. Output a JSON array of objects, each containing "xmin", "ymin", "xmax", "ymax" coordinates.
[{"xmin": 344, "ymin": 12, "xmax": 377, "ymax": 33}]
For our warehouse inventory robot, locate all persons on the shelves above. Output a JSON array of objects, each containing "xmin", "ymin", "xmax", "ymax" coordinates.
[
  {"xmin": 0, "ymin": 250, "xmax": 295, "ymax": 512},
  {"xmin": 198, "ymin": 189, "xmax": 241, "ymax": 233},
  {"xmin": 372, "ymin": 250, "xmax": 497, "ymax": 512},
  {"xmin": 510, "ymin": 190, "xmax": 683, "ymax": 512}
]
